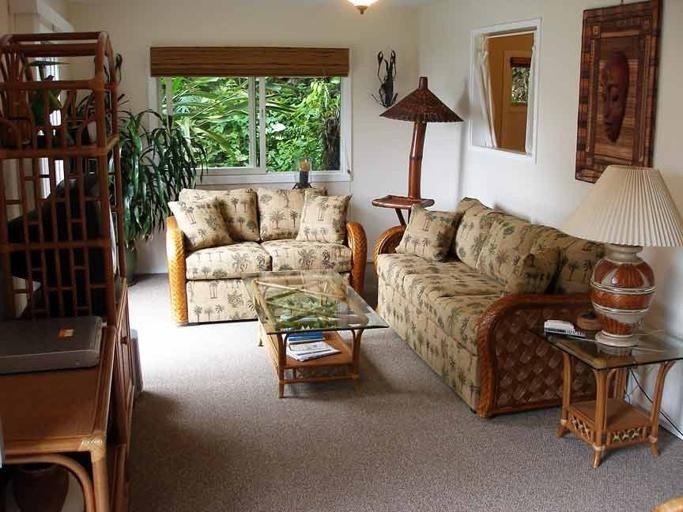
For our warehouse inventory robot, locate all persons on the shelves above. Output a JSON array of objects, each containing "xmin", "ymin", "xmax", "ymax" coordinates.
[{"xmin": 600, "ymin": 50, "xmax": 630, "ymax": 142}]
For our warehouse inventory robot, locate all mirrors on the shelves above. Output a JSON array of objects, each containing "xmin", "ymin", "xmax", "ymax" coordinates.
[{"xmin": 466, "ymin": 17, "xmax": 543, "ymax": 165}]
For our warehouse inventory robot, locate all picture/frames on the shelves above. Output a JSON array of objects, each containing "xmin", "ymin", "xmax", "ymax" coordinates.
[{"xmin": 575, "ymin": 0, "xmax": 663, "ymax": 183}]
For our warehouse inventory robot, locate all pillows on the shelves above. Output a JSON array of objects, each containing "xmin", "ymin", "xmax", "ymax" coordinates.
[
  {"xmin": 178, "ymin": 187, "xmax": 262, "ymax": 243},
  {"xmin": 295, "ymin": 190, "xmax": 353, "ymax": 243},
  {"xmin": 393, "ymin": 197, "xmax": 606, "ymax": 297},
  {"xmin": 257, "ymin": 183, "xmax": 327, "ymax": 241},
  {"xmin": 168, "ymin": 195, "xmax": 237, "ymax": 252}
]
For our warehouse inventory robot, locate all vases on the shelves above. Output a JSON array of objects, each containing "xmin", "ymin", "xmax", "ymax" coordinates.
[{"xmin": 11, "ymin": 461, "xmax": 68, "ymax": 512}]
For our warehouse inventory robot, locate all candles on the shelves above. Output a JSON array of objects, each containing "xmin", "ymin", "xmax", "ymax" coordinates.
[{"xmin": 300, "ymin": 159, "xmax": 310, "ymax": 171}]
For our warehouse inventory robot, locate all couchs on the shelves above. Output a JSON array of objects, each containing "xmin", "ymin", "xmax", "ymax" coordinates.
[
  {"xmin": 373, "ymin": 197, "xmax": 605, "ymax": 418},
  {"xmin": 165, "ymin": 186, "xmax": 368, "ymax": 325}
]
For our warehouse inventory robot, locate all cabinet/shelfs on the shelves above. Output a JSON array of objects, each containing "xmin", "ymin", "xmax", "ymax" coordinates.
[{"xmin": 0, "ymin": 31, "xmax": 137, "ymax": 457}]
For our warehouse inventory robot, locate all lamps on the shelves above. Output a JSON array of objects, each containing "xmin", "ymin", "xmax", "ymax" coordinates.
[
  {"xmin": 347, "ymin": 0, "xmax": 378, "ymax": 15},
  {"xmin": 561, "ymin": 164, "xmax": 683, "ymax": 348},
  {"xmin": 378, "ymin": 76, "xmax": 464, "ymax": 206}
]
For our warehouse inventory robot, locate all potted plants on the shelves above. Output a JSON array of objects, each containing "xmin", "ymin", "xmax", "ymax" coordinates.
[{"xmin": 0, "ymin": 44, "xmax": 238, "ymax": 288}]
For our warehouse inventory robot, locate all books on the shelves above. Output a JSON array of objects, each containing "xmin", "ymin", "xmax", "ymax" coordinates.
[{"xmin": 282, "ymin": 333, "xmax": 341, "ymax": 362}]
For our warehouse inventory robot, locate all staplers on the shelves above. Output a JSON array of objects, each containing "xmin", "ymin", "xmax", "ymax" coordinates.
[{"xmin": 543, "ymin": 320, "xmax": 574, "ymax": 335}]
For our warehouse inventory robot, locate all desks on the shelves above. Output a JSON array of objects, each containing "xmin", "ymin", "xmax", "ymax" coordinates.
[
  {"xmin": 238, "ymin": 267, "xmax": 389, "ymax": 398},
  {"xmin": 0, "ymin": 325, "xmax": 131, "ymax": 511}
]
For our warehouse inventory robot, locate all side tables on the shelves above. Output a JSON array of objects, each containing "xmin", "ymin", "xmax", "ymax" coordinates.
[
  {"xmin": 372, "ymin": 198, "xmax": 434, "ymax": 227},
  {"xmin": 527, "ymin": 327, "xmax": 683, "ymax": 469}
]
